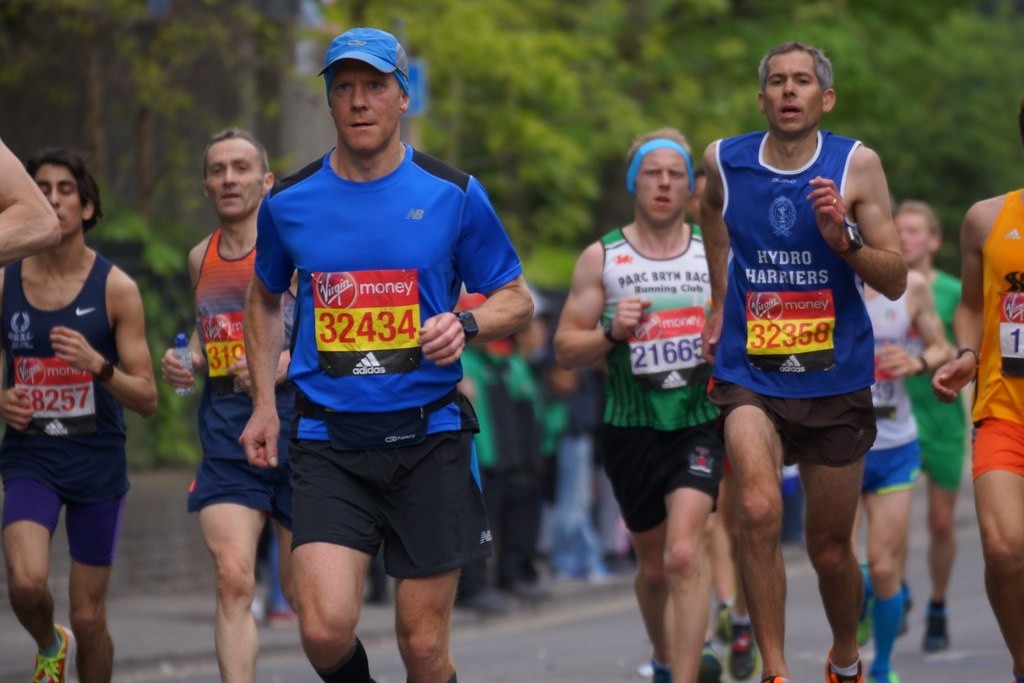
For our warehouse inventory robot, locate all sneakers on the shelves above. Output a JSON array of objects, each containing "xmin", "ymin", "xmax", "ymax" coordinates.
[
  {"xmin": 32, "ymin": 623, "xmax": 75, "ymax": 683},
  {"xmin": 760, "ymin": 676, "xmax": 793, "ymax": 683},
  {"xmin": 824, "ymin": 643, "xmax": 864, "ymax": 683}
]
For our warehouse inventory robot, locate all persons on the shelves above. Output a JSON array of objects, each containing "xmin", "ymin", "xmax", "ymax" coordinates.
[
  {"xmin": 267, "ymin": 126, "xmax": 963, "ymax": 683},
  {"xmin": 238, "ymin": 27, "xmax": 536, "ymax": 683},
  {"xmin": 700, "ymin": 42, "xmax": 909, "ymax": 683},
  {"xmin": 1, "ymin": 147, "xmax": 158, "ymax": 683},
  {"xmin": 0, "ymin": 137, "xmax": 62, "ymax": 266},
  {"xmin": 160, "ymin": 127, "xmax": 299, "ymax": 683},
  {"xmin": 930, "ymin": 93, "xmax": 1024, "ymax": 683}
]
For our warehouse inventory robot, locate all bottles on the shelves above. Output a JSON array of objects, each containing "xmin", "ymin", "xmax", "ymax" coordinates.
[{"xmin": 174, "ymin": 334, "xmax": 196, "ymax": 396}]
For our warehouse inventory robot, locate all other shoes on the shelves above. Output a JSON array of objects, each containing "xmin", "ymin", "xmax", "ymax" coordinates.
[
  {"xmin": 857, "ymin": 558, "xmax": 876, "ymax": 644},
  {"xmin": 922, "ymin": 599, "xmax": 949, "ymax": 652},
  {"xmin": 728, "ymin": 607, "xmax": 763, "ymax": 680},
  {"xmin": 701, "ymin": 646, "xmax": 723, "ymax": 683},
  {"xmin": 652, "ymin": 657, "xmax": 672, "ymax": 683},
  {"xmin": 717, "ymin": 600, "xmax": 735, "ymax": 644},
  {"xmin": 867, "ymin": 664, "xmax": 900, "ymax": 683},
  {"xmin": 900, "ymin": 584, "xmax": 912, "ymax": 633},
  {"xmin": 266, "ymin": 607, "xmax": 300, "ymax": 629}
]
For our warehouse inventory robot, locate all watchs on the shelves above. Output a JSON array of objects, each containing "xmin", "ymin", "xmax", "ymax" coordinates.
[
  {"xmin": 604, "ymin": 322, "xmax": 625, "ymax": 345},
  {"xmin": 955, "ymin": 348, "xmax": 979, "ymax": 383},
  {"xmin": 915, "ymin": 356, "xmax": 928, "ymax": 376},
  {"xmin": 453, "ymin": 312, "xmax": 479, "ymax": 342},
  {"xmin": 93, "ymin": 360, "xmax": 114, "ymax": 383},
  {"xmin": 844, "ymin": 225, "xmax": 863, "ymax": 254}
]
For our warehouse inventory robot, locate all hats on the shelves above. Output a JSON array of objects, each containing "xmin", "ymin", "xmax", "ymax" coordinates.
[{"xmin": 319, "ymin": 28, "xmax": 410, "ymax": 108}]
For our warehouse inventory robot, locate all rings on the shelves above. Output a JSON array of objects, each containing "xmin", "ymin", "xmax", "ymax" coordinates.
[{"xmin": 832, "ymin": 197, "xmax": 837, "ymax": 205}]
